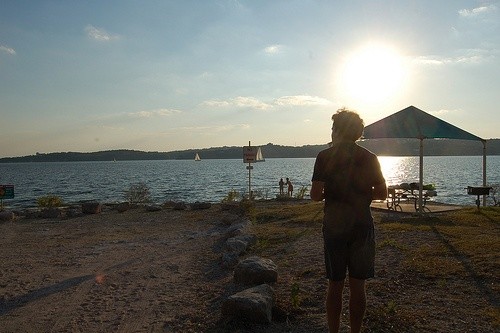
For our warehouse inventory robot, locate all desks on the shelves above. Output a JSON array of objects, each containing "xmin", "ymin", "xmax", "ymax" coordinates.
[{"xmin": 388, "ymin": 185, "xmax": 436, "ymax": 212}]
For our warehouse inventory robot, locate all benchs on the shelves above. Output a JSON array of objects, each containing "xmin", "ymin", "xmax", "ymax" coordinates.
[{"xmin": 387, "ymin": 192, "xmax": 437, "ymax": 212}]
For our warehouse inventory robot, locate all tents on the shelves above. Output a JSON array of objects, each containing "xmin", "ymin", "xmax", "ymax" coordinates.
[{"xmin": 330, "ymin": 105, "xmax": 486, "ymax": 210}]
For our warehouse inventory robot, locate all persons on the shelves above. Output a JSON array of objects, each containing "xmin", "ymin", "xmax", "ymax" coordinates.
[
  {"xmin": 285, "ymin": 178, "xmax": 293, "ymax": 196},
  {"xmin": 279, "ymin": 178, "xmax": 284, "ymax": 195},
  {"xmin": 310, "ymin": 109, "xmax": 387, "ymax": 333}
]
